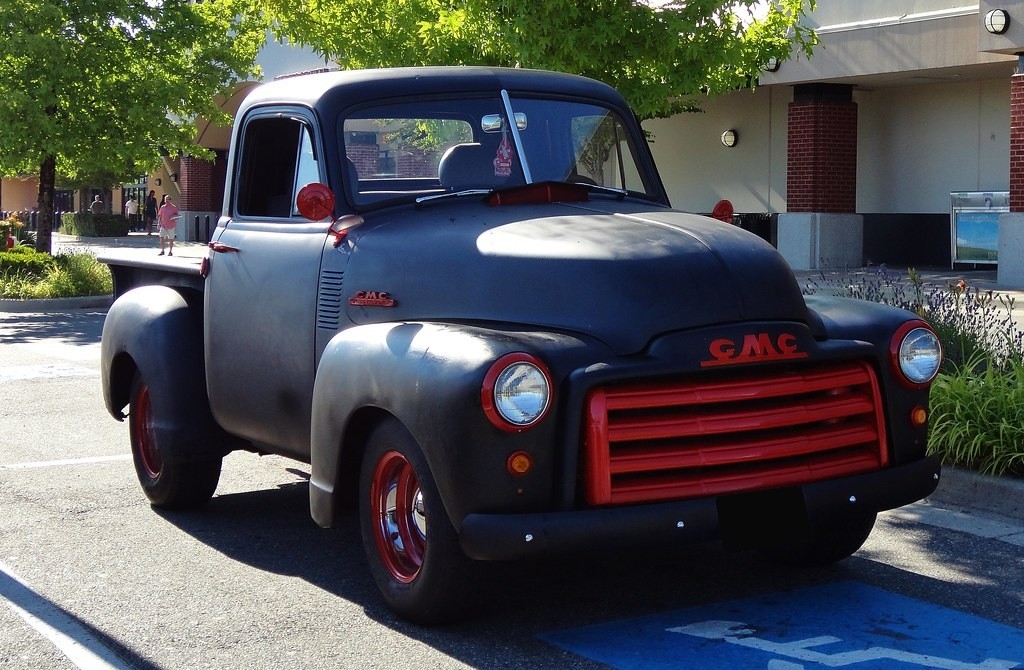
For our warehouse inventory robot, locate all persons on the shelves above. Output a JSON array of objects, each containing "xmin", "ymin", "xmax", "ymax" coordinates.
[
  {"xmin": 144, "ymin": 190, "xmax": 178, "ymax": 256},
  {"xmin": 125, "ymin": 197, "xmax": 139, "ymax": 232},
  {"xmin": 90, "ymin": 195, "xmax": 103, "ymax": 215}
]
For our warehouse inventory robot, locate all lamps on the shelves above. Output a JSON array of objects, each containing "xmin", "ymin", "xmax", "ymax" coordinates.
[
  {"xmin": 170, "ymin": 173, "xmax": 178, "ymax": 182},
  {"xmin": 721, "ymin": 129, "xmax": 737, "ymax": 147},
  {"xmin": 984, "ymin": 9, "xmax": 1010, "ymax": 35},
  {"xmin": 763, "ymin": 56, "xmax": 781, "ymax": 73},
  {"xmin": 154, "ymin": 178, "xmax": 162, "ymax": 186}
]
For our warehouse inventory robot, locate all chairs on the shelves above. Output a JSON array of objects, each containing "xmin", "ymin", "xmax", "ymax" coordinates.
[
  {"xmin": 439, "ymin": 142, "xmax": 519, "ymax": 189},
  {"xmin": 277, "ymin": 149, "xmax": 360, "ymax": 220}
]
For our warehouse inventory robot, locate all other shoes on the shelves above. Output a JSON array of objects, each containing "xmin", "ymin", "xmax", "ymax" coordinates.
[
  {"xmin": 168, "ymin": 252, "xmax": 172, "ymax": 256},
  {"xmin": 148, "ymin": 234, "xmax": 151, "ymax": 236},
  {"xmin": 158, "ymin": 252, "xmax": 165, "ymax": 255}
]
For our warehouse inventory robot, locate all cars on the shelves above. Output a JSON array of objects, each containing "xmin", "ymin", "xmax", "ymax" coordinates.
[{"xmin": 92, "ymin": 65, "xmax": 944, "ymax": 622}]
